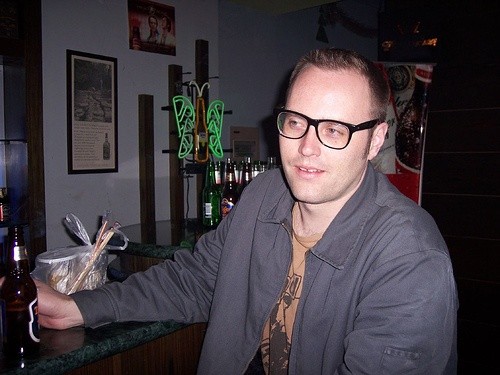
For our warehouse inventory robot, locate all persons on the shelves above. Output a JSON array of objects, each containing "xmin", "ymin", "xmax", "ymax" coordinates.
[{"xmin": 0, "ymin": 47, "xmax": 456, "ymax": 375}]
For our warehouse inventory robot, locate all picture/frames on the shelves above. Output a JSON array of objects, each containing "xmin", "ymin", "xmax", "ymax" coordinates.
[{"xmin": 66, "ymin": 49, "xmax": 119, "ymax": 173}]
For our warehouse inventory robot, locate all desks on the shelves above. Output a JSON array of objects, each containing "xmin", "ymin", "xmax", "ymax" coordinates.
[{"xmin": 1, "ymin": 219, "xmax": 215, "ymax": 374}]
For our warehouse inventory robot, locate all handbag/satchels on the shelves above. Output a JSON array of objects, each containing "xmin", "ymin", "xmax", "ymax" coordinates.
[{"xmin": 28, "ymin": 208, "xmax": 130, "ymax": 296}]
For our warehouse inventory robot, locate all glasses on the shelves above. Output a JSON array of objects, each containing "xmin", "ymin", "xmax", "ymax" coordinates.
[{"xmin": 276, "ymin": 104, "xmax": 381, "ymax": 151}]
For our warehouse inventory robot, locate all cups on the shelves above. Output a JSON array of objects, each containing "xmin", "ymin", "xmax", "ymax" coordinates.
[{"xmin": 37, "ymin": 246, "xmax": 106, "ymax": 295}]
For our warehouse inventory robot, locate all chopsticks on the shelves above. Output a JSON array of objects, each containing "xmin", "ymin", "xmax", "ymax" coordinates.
[{"xmin": 67, "ymin": 220, "xmax": 115, "ymax": 296}]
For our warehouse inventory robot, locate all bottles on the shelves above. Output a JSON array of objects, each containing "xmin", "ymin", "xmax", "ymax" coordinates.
[
  {"xmin": 0, "ymin": 225, "xmax": 42, "ymax": 366},
  {"xmin": 395, "ymin": 64, "xmax": 432, "ymax": 178},
  {"xmin": 0, "ymin": 188, "xmax": 9, "ymax": 227},
  {"xmin": 202, "ymin": 156, "xmax": 276, "ymax": 230},
  {"xmin": 103, "ymin": 133, "xmax": 110, "ymax": 160}
]
